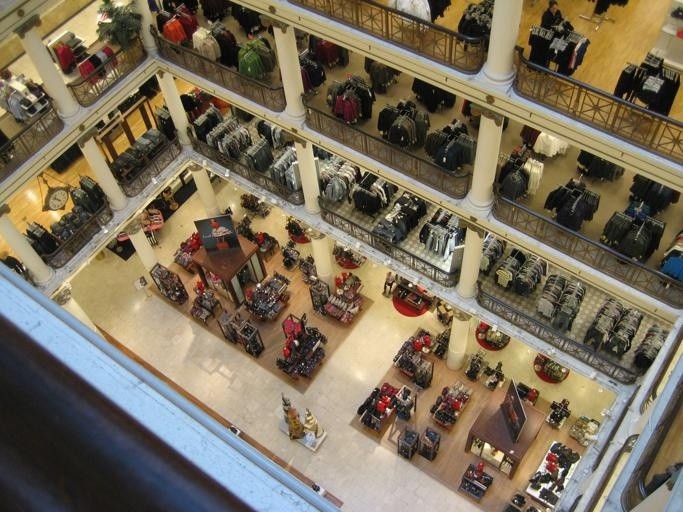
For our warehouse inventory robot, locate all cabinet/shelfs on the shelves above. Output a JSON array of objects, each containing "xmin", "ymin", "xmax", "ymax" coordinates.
[
  {"xmin": 150, "ymin": 220, "xmax": 370, "ymax": 379},
  {"xmin": 521, "ymin": 440, "xmax": 583, "ymax": 511},
  {"xmin": 393, "ymin": 328, "xmax": 450, "ymax": 389},
  {"xmin": 546, "ymin": 398, "xmax": 572, "ymax": 428},
  {"xmin": 569, "ymin": 415, "xmax": 600, "ymax": 447},
  {"xmin": 429, "ymin": 381, "xmax": 475, "ymax": 432},
  {"xmin": 396, "ymin": 426, "xmax": 420, "ymax": 461},
  {"xmin": 420, "ymin": 427, "xmax": 441, "ymax": 461},
  {"xmin": 459, "ymin": 463, "xmax": 493, "ymax": 504},
  {"xmin": 358, "ymin": 380, "xmax": 419, "ymax": 431},
  {"xmin": 467, "ymin": 347, "xmax": 506, "ymax": 391},
  {"xmin": 507, "ymin": 486, "xmax": 550, "ymax": 511},
  {"xmin": 518, "ymin": 381, "xmax": 541, "ymax": 406}
]
